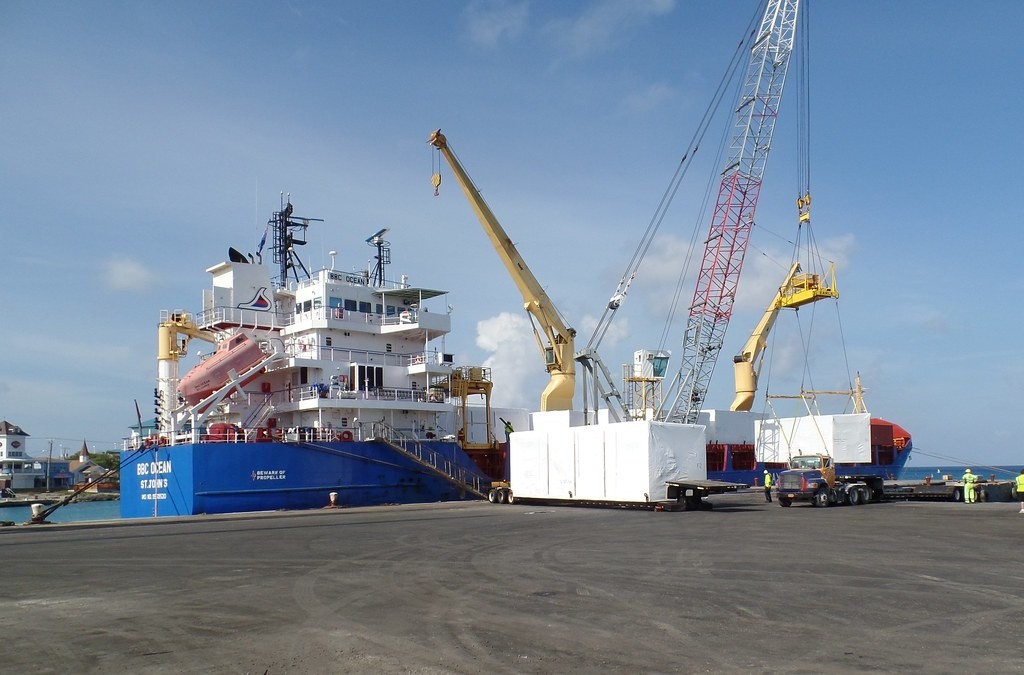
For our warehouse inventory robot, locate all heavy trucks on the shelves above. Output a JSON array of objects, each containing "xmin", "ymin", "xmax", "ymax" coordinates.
[{"xmin": 774, "ymin": 449, "xmax": 1020, "ymax": 506}]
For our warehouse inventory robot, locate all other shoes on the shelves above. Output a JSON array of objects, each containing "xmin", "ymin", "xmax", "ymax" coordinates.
[{"xmin": 1019, "ymin": 509, "xmax": 1024, "ymax": 513}]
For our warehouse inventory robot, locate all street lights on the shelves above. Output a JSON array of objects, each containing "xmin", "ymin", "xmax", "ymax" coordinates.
[
  {"xmin": 46, "ymin": 441, "xmax": 53, "ymax": 490},
  {"xmin": 60, "ymin": 445, "xmax": 63, "ymax": 459},
  {"xmin": 114, "ymin": 443, "xmax": 116, "ymax": 449},
  {"xmin": 93, "ymin": 446, "xmax": 95, "ymax": 454}
]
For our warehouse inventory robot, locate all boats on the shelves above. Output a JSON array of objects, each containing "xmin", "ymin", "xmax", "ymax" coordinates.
[
  {"xmin": 120, "ymin": 195, "xmax": 914, "ymax": 518},
  {"xmin": 177, "ymin": 333, "xmax": 267, "ymax": 413}
]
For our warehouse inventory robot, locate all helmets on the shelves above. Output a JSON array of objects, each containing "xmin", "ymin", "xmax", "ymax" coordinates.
[
  {"xmin": 764, "ymin": 470, "xmax": 768, "ymax": 473},
  {"xmin": 965, "ymin": 469, "xmax": 971, "ymax": 473}
]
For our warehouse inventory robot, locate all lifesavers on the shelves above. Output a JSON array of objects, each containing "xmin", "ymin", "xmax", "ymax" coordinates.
[{"xmin": 400, "ymin": 311, "xmax": 411, "ymax": 320}]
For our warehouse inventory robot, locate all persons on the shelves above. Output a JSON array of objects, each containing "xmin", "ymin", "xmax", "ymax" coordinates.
[
  {"xmin": 963, "ymin": 468, "xmax": 977, "ymax": 504},
  {"xmin": 504, "ymin": 421, "xmax": 513, "ymax": 442},
  {"xmin": 763, "ymin": 470, "xmax": 772, "ymax": 503},
  {"xmin": 1015, "ymin": 470, "xmax": 1024, "ymax": 514}
]
